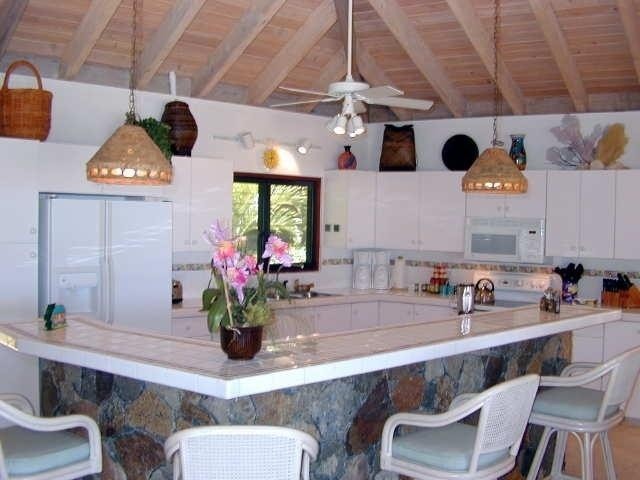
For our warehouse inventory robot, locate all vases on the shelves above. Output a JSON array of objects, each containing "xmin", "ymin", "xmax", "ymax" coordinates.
[
  {"xmin": 162, "ymin": 101, "xmax": 198, "ymax": 156},
  {"xmin": 338, "ymin": 145, "xmax": 356, "ymax": 169},
  {"xmin": 508, "ymin": 133, "xmax": 526, "ymax": 170}
]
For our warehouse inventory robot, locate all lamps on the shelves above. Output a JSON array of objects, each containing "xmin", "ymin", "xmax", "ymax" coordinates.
[
  {"xmin": 462, "ymin": 0, "xmax": 528, "ymax": 194},
  {"xmin": 86, "ymin": 1, "xmax": 173, "ymax": 185},
  {"xmin": 214, "ymin": 132, "xmax": 323, "ymax": 155},
  {"xmin": 327, "ymin": 95, "xmax": 366, "ymax": 138}
]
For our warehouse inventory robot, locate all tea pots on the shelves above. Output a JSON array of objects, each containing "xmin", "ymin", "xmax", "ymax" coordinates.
[{"xmin": 475, "ymin": 278, "xmax": 494, "ymax": 305}]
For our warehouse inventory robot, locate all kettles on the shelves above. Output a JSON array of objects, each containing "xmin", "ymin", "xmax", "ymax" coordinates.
[{"xmin": 390, "ymin": 256, "xmax": 408, "ymax": 292}]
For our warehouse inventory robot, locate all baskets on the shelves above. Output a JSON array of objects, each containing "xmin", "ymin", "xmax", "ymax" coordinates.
[{"xmin": 0, "ymin": 61, "xmax": 53, "ymax": 142}]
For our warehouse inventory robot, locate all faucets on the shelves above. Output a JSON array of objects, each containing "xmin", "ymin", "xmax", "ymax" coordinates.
[{"xmin": 276, "ymin": 263, "xmax": 304, "ymax": 281}]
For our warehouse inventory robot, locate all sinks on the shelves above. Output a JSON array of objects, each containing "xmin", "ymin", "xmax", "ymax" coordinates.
[{"xmin": 289, "ymin": 291, "xmax": 339, "ymax": 299}]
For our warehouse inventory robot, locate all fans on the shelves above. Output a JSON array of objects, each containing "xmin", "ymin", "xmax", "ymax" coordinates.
[{"xmin": 270, "ymin": 0, "xmax": 434, "ymax": 113}]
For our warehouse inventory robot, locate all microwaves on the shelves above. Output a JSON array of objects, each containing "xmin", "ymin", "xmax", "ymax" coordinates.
[{"xmin": 463, "ymin": 216, "xmax": 544, "ymax": 263}]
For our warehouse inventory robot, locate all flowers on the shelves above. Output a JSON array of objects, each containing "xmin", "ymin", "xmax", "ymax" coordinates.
[
  {"xmin": 544, "ymin": 115, "xmax": 631, "ymax": 171},
  {"xmin": 198, "ymin": 219, "xmax": 293, "ymax": 337}
]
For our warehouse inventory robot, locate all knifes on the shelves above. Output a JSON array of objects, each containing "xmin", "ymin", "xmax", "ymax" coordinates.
[{"xmin": 615, "ymin": 273, "xmax": 631, "ymax": 288}]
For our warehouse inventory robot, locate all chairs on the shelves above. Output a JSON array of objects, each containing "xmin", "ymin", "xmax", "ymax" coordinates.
[
  {"xmin": 220, "ymin": 323, "xmax": 263, "ymax": 360},
  {"xmin": 528, "ymin": 346, "xmax": 640, "ymax": 480},
  {"xmin": 380, "ymin": 373, "xmax": 540, "ymax": 480},
  {"xmin": 0, "ymin": 394, "xmax": 103, "ymax": 480},
  {"xmin": 164, "ymin": 425, "xmax": 319, "ymax": 480}
]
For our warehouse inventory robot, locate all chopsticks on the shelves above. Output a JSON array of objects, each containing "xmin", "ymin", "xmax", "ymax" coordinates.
[{"xmin": 602, "ymin": 278, "xmax": 619, "ymax": 293}]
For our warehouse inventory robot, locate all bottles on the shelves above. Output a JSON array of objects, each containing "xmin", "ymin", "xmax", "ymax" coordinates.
[{"xmin": 414, "ymin": 283, "xmax": 418, "ymax": 291}]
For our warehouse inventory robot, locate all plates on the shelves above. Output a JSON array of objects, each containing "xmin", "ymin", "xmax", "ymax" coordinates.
[{"xmin": 441, "ymin": 134, "xmax": 479, "ymax": 171}]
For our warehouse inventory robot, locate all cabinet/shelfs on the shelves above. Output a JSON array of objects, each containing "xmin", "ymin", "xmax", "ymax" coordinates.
[
  {"xmin": 170, "ymin": 156, "xmax": 235, "ymax": 253},
  {"xmin": 324, "ymin": 170, "xmax": 375, "ymax": 249},
  {"xmin": 614, "ymin": 171, "xmax": 640, "ymax": 259},
  {"xmin": 572, "ymin": 321, "xmax": 640, "ymax": 427},
  {"xmin": 545, "ymin": 171, "xmax": 614, "ymax": 260},
  {"xmin": 466, "ymin": 171, "xmax": 546, "ymax": 216},
  {"xmin": 173, "ymin": 300, "xmax": 458, "ymax": 340},
  {"xmin": 375, "ymin": 172, "xmax": 466, "ymax": 253}
]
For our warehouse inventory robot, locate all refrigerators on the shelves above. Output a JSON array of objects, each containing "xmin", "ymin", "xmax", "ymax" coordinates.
[{"xmin": 39, "ymin": 194, "xmax": 174, "ymax": 333}]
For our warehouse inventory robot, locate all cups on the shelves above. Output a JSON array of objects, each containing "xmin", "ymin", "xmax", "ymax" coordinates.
[
  {"xmin": 457, "ymin": 284, "xmax": 474, "ymax": 314},
  {"xmin": 457, "ymin": 315, "xmax": 472, "ymax": 335},
  {"xmin": 441, "ymin": 286, "xmax": 447, "ymax": 295}
]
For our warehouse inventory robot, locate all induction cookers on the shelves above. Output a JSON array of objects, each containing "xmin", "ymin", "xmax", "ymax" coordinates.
[{"xmin": 494, "ymin": 299, "xmax": 536, "ymax": 307}]
